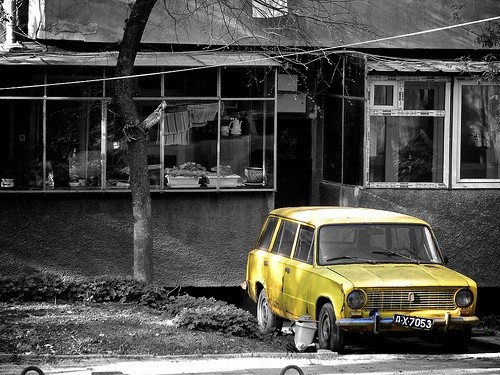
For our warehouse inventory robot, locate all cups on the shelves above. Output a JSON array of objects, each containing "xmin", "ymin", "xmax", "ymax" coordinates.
[{"xmin": 221, "ymin": 126, "xmax": 229, "ymax": 135}]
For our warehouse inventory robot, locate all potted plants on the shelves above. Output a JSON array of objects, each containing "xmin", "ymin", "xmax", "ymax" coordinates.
[
  {"xmin": 204, "ymin": 165, "xmax": 240, "ymax": 188},
  {"xmin": 164, "ymin": 161, "xmax": 207, "ymax": 188}
]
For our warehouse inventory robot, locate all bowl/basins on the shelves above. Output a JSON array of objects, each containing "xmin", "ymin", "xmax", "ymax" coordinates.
[{"xmin": 244, "ymin": 166, "xmax": 263, "ymax": 183}]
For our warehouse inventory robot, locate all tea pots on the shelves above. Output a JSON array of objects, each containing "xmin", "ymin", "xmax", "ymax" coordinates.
[{"xmin": 229, "ymin": 118, "xmax": 242, "ymax": 134}]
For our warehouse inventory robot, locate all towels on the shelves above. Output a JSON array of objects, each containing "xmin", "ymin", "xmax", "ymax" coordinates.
[{"xmin": 156, "ymin": 102, "xmax": 224, "ymax": 146}]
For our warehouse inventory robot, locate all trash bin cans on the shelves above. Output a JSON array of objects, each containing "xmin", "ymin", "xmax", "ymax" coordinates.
[{"xmin": 294, "ymin": 319, "xmax": 318, "ymax": 349}]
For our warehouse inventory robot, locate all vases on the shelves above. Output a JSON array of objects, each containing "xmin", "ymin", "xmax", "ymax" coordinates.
[{"xmin": 244, "ymin": 167, "xmax": 262, "ymax": 183}]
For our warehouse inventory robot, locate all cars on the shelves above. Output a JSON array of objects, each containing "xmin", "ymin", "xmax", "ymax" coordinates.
[{"xmin": 245, "ymin": 205, "xmax": 480, "ymax": 352}]
